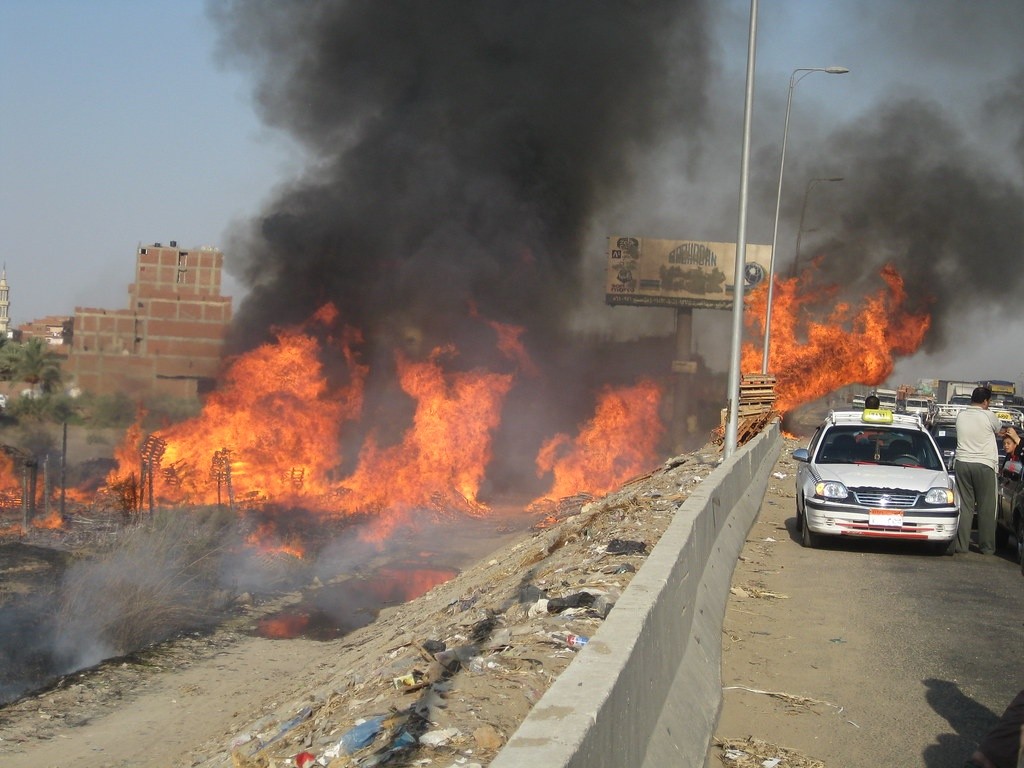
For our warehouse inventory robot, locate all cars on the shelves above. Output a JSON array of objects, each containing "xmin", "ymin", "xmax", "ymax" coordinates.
[
  {"xmin": 793, "ymin": 409, "xmax": 960, "ymax": 558},
  {"xmin": 849, "ymin": 372, "xmax": 1024, "ymax": 573}
]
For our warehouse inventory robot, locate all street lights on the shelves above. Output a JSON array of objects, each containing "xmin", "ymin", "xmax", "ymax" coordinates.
[
  {"xmin": 791, "ymin": 173, "xmax": 845, "ymax": 279},
  {"xmin": 761, "ymin": 64, "xmax": 850, "ymax": 376}
]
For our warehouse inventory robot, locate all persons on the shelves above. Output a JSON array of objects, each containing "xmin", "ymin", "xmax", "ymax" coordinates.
[
  {"xmin": 853, "ymin": 395, "xmax": 886, "ymax": 459},
  {"xmin": 1005, "ymin": 427, "xmax": 1024, "ymax": 451},
  {"xmin": 953, "ymin": 387, "xmax": 1007, "ymax": 556},
  {"xmin": 998, "ymin": 432, "xmax": 1024, "ymax": 483}
]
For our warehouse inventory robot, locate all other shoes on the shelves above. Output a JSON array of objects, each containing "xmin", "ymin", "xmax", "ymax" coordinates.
[
  {"xmin": 955, "ymin": 547, "xmax": 969, "ymax": 553},
  {"xmin": 976, "ymin": 546, "xmax": 995, "ymax": 555}
]
